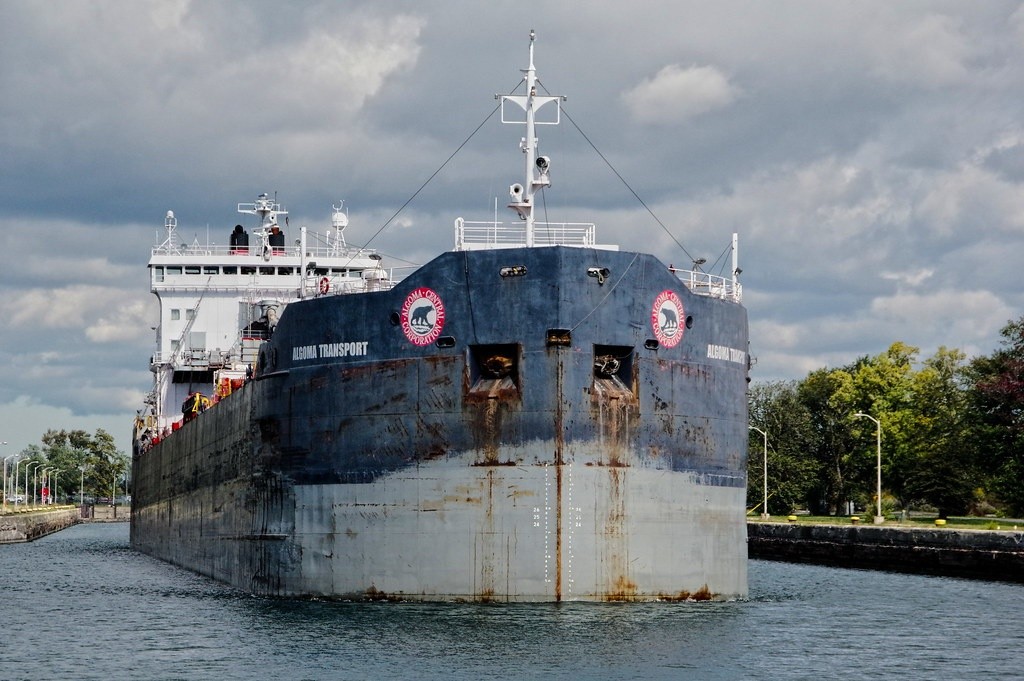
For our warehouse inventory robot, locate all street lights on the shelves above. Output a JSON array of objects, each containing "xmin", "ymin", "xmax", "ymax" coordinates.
[
  {"xmin": 853, "ymin": 412, "xmax": 881, "ymax": 518},
  {"xmin": 747, "ymin": 425, "xmax": 768, "ymax": 513},
  {"xmin": 2, "ymin": 453, "xmax": 67, "ymax": 509}
]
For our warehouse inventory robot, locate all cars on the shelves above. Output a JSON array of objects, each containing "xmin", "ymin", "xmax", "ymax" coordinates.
[{"xmin": 2, "ymin": 492, "xmax": 132, "ymax": 505}]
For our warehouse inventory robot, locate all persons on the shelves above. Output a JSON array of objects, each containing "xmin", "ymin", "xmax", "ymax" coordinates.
[{"xmin": 138, "ymin": 426, "xmax": 173, "ymax": 459}]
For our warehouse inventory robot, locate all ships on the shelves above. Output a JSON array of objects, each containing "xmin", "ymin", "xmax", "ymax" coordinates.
[{"xmin": 128, "ymin": 25, "xmax": 754, "ymax": 604}]
